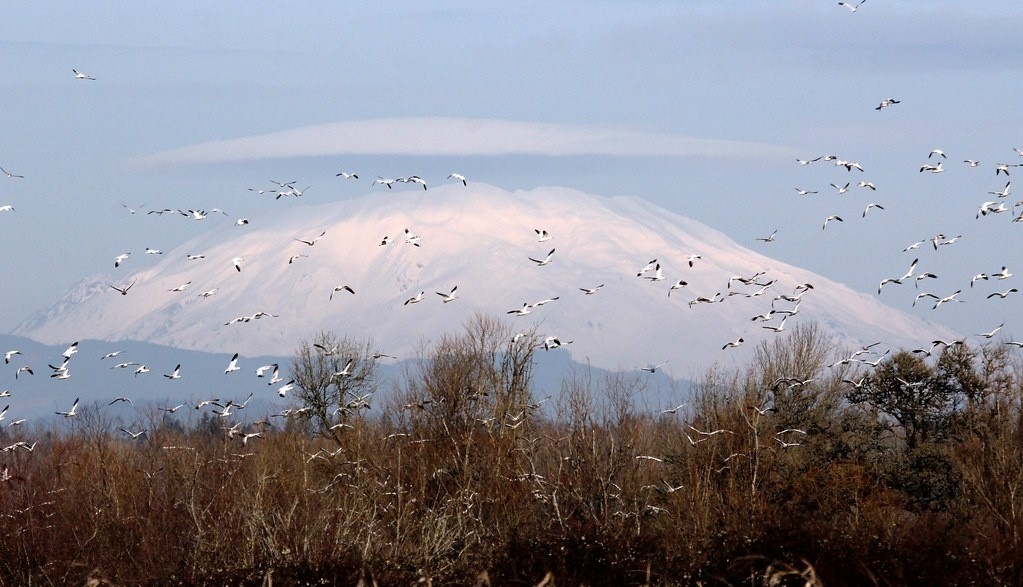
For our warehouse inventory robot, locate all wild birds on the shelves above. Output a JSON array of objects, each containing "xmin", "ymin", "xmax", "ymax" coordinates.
[
  {"xmin": 71, "ymin": 67, "xmax": 97, "ymax": 81},
  {"xmin": 1, "ymin": 141, "xmax": 1022, "ymax": 587},
  {"xmin": 837, "ymin": 0, "xmax": 866, "ymax": 13},
  {"xmin": 875, "ymin": 97, "xmax": 901, "ymax": 111}
]
